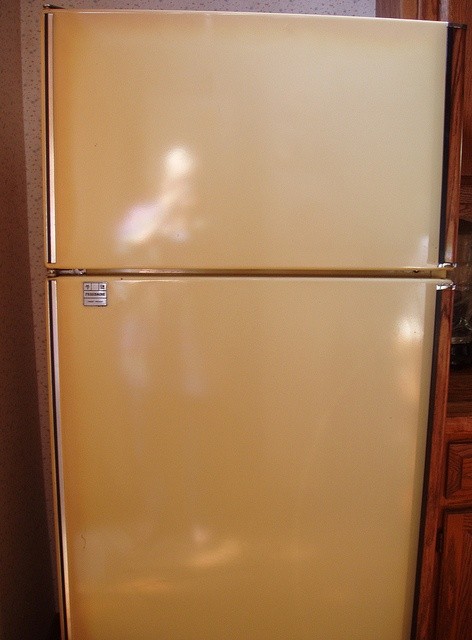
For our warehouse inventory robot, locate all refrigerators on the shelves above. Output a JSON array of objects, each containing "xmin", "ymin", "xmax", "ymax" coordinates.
[{"xmin": 40, "ymin": 10, "xmax": 450, "ymax": 630}]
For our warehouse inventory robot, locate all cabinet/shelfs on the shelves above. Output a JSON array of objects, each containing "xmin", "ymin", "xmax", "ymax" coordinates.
[{"xmin": 411, "ymin": 0, "xmax": 471, "ymax": 640}]
